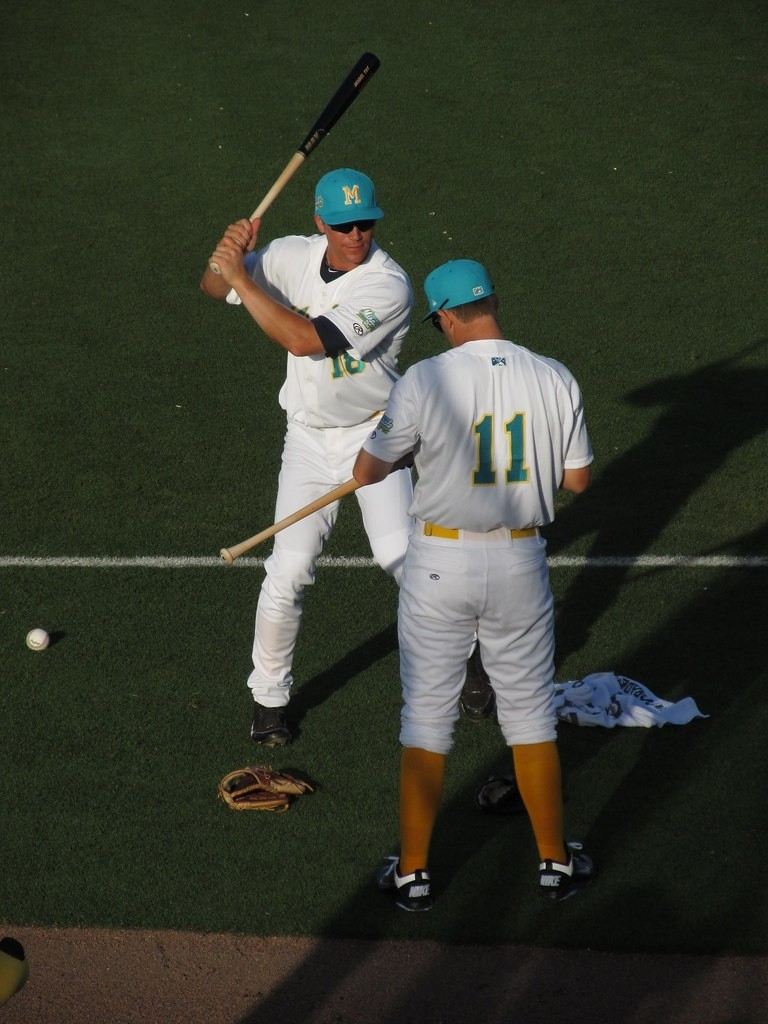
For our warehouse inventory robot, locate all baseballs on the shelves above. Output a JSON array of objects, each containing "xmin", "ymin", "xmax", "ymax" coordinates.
[{"xmin": 23, "ymin": 628, "xmax": 51, "ymax": 652}]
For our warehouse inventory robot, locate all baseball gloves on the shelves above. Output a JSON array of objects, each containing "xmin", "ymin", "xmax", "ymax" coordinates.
[{"xmin": 215, "ymin": 763, "xmax": 317, "ymax": 816}]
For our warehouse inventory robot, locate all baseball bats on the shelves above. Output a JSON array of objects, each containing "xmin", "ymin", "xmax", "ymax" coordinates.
[
  {"xmin": 204, "ymin": 50, "xmax": 383, "ymax": 277},
  {"xmin": 216, "ymin": 449, "xmax": 420, "ymax": 565}
]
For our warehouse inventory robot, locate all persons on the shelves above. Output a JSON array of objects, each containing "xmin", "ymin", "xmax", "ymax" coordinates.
[
  {"xmin": 353, "ymin": 259, "xmax": 595, "ymax": 912},
  {"xmin": 200, "ymin": 168, "xmax": 496, "ymax": 746}
]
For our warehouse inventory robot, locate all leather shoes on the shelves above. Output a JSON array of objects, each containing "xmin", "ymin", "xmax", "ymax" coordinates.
[{"xmin": 459, "ymin": 660, "xmax": 495, "ymax": 723}]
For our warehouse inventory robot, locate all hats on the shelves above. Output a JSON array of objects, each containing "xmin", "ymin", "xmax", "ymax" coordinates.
[
  {"xmin": 420, "ymin": 259, "xmax": 495, "ymax": 323},
  {"xmin": 315, "ymin": 168, "xmax": 385, "ymax": 225}
]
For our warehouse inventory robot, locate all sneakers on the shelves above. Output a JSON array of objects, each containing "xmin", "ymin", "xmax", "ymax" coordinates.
[
  {"xmin": 250, "ymin": 702, "xmax": 291, "ymax": 747},
  {"xmin": 376, "ymin": 856, "xmax": 433, "ymax": 911},
  {"xmin": 537, "ymin": 840, "xmax": 583, "ymax": 901}
]
